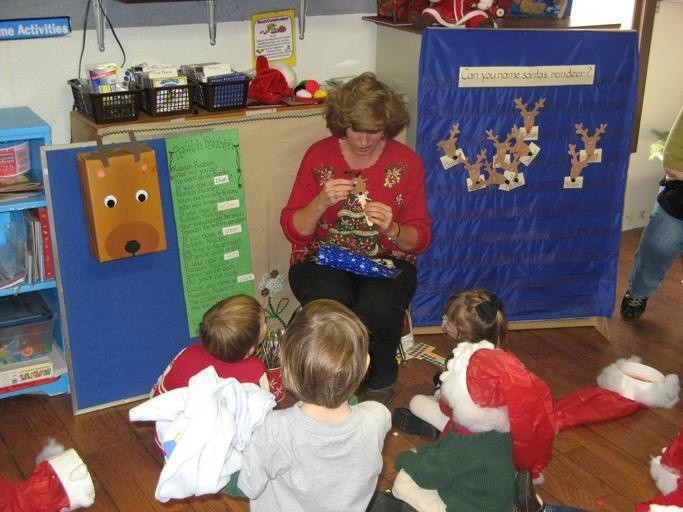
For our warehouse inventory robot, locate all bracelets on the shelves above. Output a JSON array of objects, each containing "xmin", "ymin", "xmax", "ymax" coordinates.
[{"xmin": 387, "ymin": 220, "xmax": 401, "ymax": 242}]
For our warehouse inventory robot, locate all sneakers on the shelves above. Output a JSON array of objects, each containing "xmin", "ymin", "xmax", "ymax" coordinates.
[
  {"xmin": 515, "ymin": 467, "xmax": 544, "ymax": 512},
  {"xmin": 621, "ymin": 290, "xmax": 648, "ymax": 320},
  {"xmin": 363, "ymin": 382, "xmax": 395, "ymax": 407},
  {"xmin": 366, "ymin": 494, "xmax": 419, "ymax": 512},
  {"xmin": 393, "ymin": 408, "xmax": 438, "ymax": 443}
]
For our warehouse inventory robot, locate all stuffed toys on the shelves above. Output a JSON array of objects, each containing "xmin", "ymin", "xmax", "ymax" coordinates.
[{"xmin": 245, "ymin": 55, "xmax": 326, "ymax": 105}]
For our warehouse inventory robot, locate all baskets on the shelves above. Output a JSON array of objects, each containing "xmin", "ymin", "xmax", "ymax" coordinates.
[
  {"xmin": 179, "ymin": 64, "xmax": 253, "ymax": 112},
  {"xmin": 67, "ymin": 80, "xmax": 142, "ymax": 122},
  {"xmin": 137, "ymin": 84, "xmax": 197, "ymax": 115}
]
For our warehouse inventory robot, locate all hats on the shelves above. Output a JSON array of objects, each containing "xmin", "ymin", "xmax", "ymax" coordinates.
[
  {"xmin": 440, "ymin": 340, "xmax": 554, "ymax": 485},
  {"xmin": 553, "ymin": 355, "xmax": 680, "ymax": 432},
  {"xmin": 0, "ymin": 449, "xmax": 97, "ymax": 511},
  {"xmin": 635, "ymin": 433, "xmax": 683, "ymax": 512}
]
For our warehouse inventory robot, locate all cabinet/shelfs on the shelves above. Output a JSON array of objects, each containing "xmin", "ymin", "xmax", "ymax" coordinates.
[{"xmin": 0, "ymin": 106, "xmax": 71, "ymax": 400}]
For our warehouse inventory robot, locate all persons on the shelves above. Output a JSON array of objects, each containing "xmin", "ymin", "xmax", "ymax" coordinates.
[
  {"xmin": 149, "ymin": 293, "xmax": 275, "ymax": 465},
  {"xmin": 367, "ymin": 340, "xmax": 548, "ymax": 512},
  {"xmin": 235, "ymin": 300, "xmax": 393, "ymax": 511},
  {"xmin": 278, "ymin": 68, "xmax": 434, "ymax": 391},
  {"xmin": 405, "ymin": 0, "xmax": 497, "ymax": 31},
  {"xmin": 619, "ymin": 107, "xmax": 681, "ymax": 320},
  {"xmin": 391, "ymin": 289, "xmax": 507, "ymax": 443}
]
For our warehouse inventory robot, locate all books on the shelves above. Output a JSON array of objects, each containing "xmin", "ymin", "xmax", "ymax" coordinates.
[
  {"xmin": 0, "ymin": 206, "xmax": 53, "ymax": 289},
  {"xmin": 86, "ymin": 64, "xmax": 234, "ymax": 112}
]
